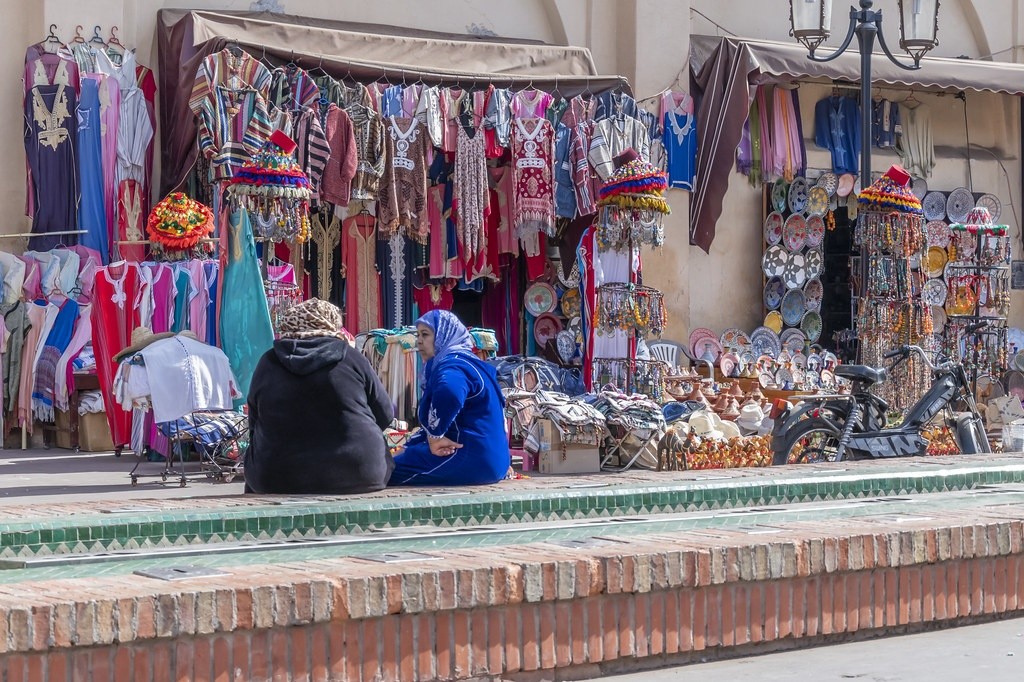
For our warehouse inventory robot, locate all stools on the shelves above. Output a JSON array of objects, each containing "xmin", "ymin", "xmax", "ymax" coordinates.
[
  {"xmin": 507, "ymin": 417, "xmax": 539, "ymax": 472},
  {"xmin": 600, "ymin": 421, "xmax": 663, "ymax": 472}
]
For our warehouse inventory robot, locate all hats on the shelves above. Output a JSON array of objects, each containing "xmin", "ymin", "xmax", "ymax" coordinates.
[{"xmin": 113, "ymin": 326, "xmax": 176, "ymax": 362}]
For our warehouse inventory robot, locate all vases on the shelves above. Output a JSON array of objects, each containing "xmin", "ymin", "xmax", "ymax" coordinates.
[
  {"xmin": 672, "ymin": 379, "xmax": 768, "ymax": 421},
  {"xmin": 699, "ymin": 339, "xmax": 854, "ymax": 378}
]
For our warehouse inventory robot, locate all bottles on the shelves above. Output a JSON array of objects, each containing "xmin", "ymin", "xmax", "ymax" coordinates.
[
  {"xmin": 669, "ymin": 338, "xmax": 854, "ymax": 419},
  {"xmin": 1013, "ymin": 347, "xmax": 1018, "ymax": 354},
  {"xmin": 1008, "ymin": 343, "xmax": 1015, "ymax": 352}
]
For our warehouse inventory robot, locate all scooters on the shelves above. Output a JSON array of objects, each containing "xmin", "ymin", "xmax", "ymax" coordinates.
[{"xmin": 771, "ymin": 321, "xmax": 993, "ymax": 465}]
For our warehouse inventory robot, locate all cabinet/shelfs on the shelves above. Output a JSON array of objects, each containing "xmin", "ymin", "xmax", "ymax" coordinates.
[
  {"xmin": 696, "ymin": 367, "xmax": 820, "ymax": 417},
  {"xmin": 822, "ymin": 225, "xmax": 859, "ymax": 336}
]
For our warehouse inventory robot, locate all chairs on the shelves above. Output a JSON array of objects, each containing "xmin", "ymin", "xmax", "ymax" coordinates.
[{"xmin": 645, "ymin": 339, "xmax": 715, "ymax": 389}]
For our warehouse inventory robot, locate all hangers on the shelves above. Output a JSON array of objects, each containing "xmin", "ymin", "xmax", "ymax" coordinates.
[
  {"xmin": 900, "ymin": 88, "xmax": 920, "ymax": 104},
  {"xmin": 107, "ymin": 166, "xmax": 213, "ymax": 276},
  {"xmin": 357, "ymin": 200, "xmax": 375, "ymax": 227},
  {"xmin": 317, "ymin": 198, "xmax": 332, "ymax": 215},
  {"xmin": 873, "ymin": 86, "xmax": 884, "ymax": 100},
  {"xmin": 0, "ymin": 230, "xmax": 90, "ymax": 306},
  {"xmin": 37, "ymin": 23, "xmax": 143, "ymax": 95},
  {"xmin": 269, "ymin": 250, "xmax": 286, "ymax": 264},
  {"xmin": 229, "ymin": 39, "xmax": 687, "ymax": 145}
]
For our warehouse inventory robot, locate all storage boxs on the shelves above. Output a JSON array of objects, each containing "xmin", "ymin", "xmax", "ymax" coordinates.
[
  {"xmin": 77, "ymin": 412, "xmax": 116, "ymax": 451},
  {"xmin": 545, "ymin": 339, "xmax": 583, "ymax": 371},
  {"xmin": 537, "ymin": 419, "xmax": 601, "ymax": 474}
]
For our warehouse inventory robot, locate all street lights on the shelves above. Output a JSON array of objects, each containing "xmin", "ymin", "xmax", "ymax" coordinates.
[{"xmin": 785, "ymin": 0, "xmax": 945, "ymax": 290}]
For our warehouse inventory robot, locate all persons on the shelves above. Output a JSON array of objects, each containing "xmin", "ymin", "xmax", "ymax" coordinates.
[
  {"xmin": 387, "ymin": 309, "xmax": 510, "ymax": 487},
  {"xmin": 243, "ymin": 297, "xmax": 395, "ymax": 495}
]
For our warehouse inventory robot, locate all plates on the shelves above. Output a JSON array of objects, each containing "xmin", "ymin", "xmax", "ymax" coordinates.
[
  {"xmin": 910, "ymin": 179, "xmax": 1002, "ymax": 332},
  {"xmin": 762, "ymin": 171, "xmax": 873, "ymax": 344},
  {"xmin": 523, "ymin": 282, "xmax": 583, "ymax": 363},
  {"xmin": 977, "ymin": 326, "xmax": 1024, "ymax": 407},
  {"xmin": 688, "ymin": 327, "xmax": 838, "ymax": 390}
]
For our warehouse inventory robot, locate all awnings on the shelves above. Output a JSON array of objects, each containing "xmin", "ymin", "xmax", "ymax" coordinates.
[
  {"xmin": 688, "ymin": 34, "xmax": 1024, "ymax": 254},
  {"xmin": 157, "ymin": 7, "xmax": 635, "ymax": 202}
]
